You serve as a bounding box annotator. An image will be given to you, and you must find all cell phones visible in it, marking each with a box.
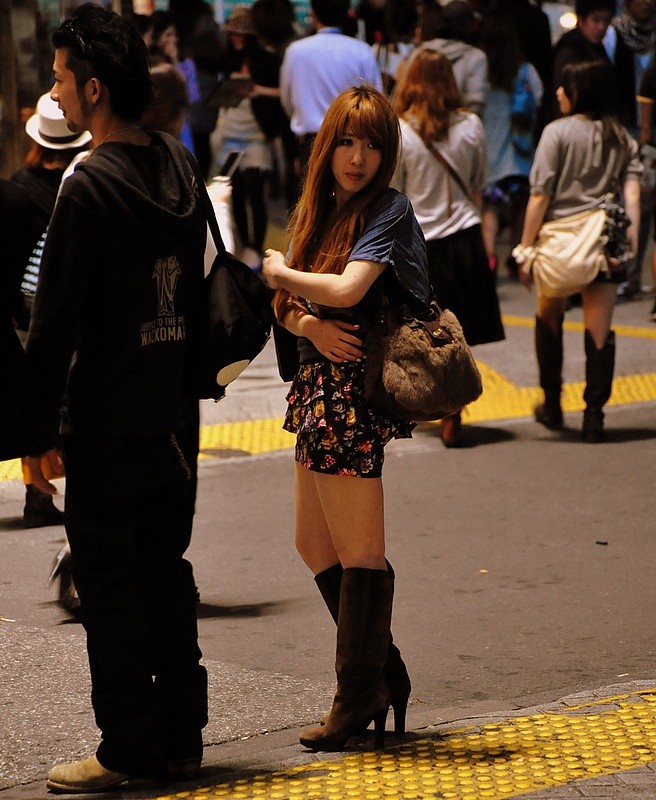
[212,150,244,182]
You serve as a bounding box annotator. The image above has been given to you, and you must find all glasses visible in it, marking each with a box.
[61,19,92,58]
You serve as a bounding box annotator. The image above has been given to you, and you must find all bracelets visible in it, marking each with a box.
[513,243,533,263]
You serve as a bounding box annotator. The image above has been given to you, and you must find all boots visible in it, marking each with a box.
[582,327,616,439]
[297,560,393,748]
[534,312,564,426]
[319,557,411,749]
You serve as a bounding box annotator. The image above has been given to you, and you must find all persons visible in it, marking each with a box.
[389,45,505,447]
[0,0,300,791]
[351,0,656,325]
[261,81,429,750]
[280,0,382,209]
[512,55,645,441]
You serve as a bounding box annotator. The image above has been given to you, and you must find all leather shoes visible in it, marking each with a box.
[47,751,198,792]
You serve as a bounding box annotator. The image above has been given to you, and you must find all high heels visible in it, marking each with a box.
[47,547,81,618]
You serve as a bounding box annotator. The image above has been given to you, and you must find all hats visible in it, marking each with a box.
[25,92,93,149]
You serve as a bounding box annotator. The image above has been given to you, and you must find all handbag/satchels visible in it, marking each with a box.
[181,146,273,401]
[532,211,609,298]
[511,63,540,157]
[365,192,483,421]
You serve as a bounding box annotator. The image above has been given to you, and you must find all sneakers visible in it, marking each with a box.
[441,420,461,445]
[22,497,67,528]
